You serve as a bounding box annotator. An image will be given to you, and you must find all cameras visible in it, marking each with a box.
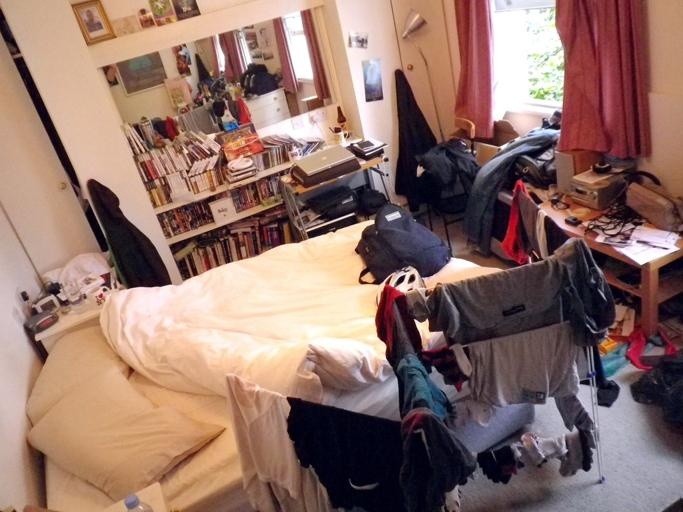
[565,215,582,226]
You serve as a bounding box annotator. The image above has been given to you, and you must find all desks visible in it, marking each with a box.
[536,193,683,343]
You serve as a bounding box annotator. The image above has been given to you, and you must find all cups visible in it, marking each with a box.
[85,285,112,309]
[333,128,349,144]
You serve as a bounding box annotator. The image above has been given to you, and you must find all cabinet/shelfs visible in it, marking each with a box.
[155,158,298,247]
[243,86,292,132]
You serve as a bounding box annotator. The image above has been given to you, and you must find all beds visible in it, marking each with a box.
[42,222,507,512]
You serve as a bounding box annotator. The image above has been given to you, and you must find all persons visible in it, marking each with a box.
[82,8,103,33]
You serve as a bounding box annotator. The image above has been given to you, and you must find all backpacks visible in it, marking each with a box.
[355,204,451,284]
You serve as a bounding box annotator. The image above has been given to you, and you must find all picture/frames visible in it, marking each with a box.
[110,51,170,98]
[72,1,119,46]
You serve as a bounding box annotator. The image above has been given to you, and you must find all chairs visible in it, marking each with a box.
[429,118,479,258]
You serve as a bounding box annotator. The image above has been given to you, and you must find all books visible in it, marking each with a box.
[122,115,327,206]
[158,203,291,281]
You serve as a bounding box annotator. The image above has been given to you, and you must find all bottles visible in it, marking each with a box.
[20,290,35,319]
[336,105,351,138]
[61,272,87,314]
[124,494,154,512]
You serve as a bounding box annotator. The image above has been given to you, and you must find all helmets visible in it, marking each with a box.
[376,266,425,307]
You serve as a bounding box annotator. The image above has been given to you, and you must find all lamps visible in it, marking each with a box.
[401,8,446,144]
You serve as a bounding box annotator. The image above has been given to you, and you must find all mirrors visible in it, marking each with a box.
[103,0,340,134]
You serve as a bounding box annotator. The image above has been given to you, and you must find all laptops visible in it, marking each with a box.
[294,144,356,176]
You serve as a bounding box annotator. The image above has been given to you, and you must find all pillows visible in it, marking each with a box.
[25,320,230,506]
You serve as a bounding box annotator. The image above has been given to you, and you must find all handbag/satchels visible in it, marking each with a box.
[358,188,388,216]
[515,154,549,190]
[625,180,683,231]
